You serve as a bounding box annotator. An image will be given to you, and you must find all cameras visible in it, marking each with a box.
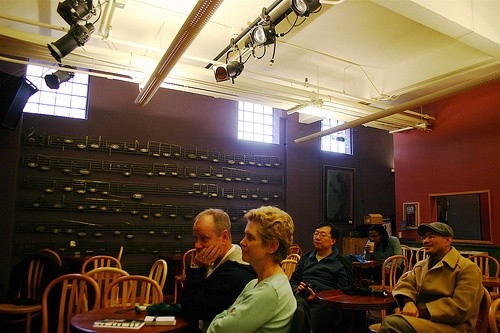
[298,282,317,299]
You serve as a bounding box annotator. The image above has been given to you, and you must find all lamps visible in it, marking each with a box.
[47,20,94,63]
[213,43,244,85]
[249,15,275,46]
[289,0,322,18]
[45,63,77,90]
[56,0,96,26]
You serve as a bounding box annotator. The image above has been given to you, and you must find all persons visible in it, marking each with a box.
[176,208,258,321]
[361,224,405,286]
[289,222,356,333]
[206,205,297,333]
[379,222,483,333]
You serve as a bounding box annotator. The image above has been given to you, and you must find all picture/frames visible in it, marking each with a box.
[428,189,494,245]
[323,165,354,224]
[402,202,420,229]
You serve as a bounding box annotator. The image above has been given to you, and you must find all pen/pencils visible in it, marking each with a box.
[153,315,157,322]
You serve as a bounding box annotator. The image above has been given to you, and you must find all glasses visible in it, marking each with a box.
[420,234,448,242]
[313,230,332,239]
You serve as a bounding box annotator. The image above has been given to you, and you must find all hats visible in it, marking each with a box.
[417,222,454,237]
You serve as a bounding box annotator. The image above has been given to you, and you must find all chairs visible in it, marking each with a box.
[0,245,500,333]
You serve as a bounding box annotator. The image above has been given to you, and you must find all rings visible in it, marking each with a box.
[202,255,206,259]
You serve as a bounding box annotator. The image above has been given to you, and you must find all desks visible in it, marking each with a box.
[351,260,378,288]
[314,288,398,333]
[70,306,186,333]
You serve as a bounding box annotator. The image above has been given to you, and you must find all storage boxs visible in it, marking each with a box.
[364,214,383,224]
